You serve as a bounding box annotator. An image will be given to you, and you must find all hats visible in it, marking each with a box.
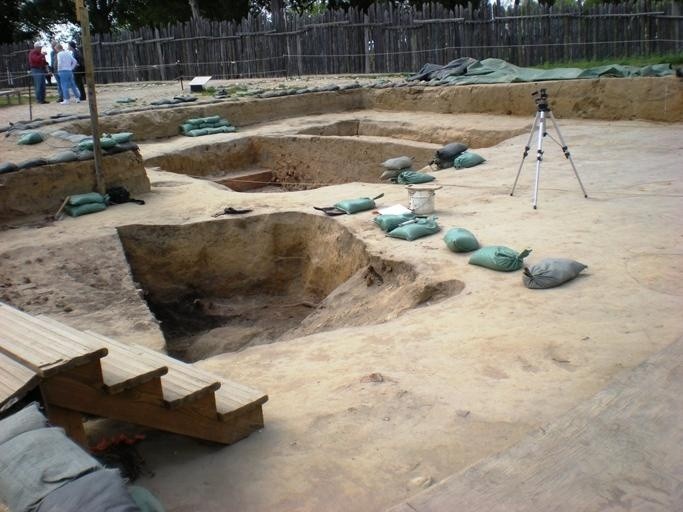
[33,42,44,48]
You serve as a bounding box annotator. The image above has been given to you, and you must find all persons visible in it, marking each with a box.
[27,41,51,105]
[54,43,81,106]
[48,41,71,103]
[66,41,86,101]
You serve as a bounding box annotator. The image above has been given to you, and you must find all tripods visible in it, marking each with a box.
[510,88,587,210]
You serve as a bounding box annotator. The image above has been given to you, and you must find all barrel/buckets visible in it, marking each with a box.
[408,188,436,215]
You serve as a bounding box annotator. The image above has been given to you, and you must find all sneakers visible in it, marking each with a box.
[36,97,86,106]
